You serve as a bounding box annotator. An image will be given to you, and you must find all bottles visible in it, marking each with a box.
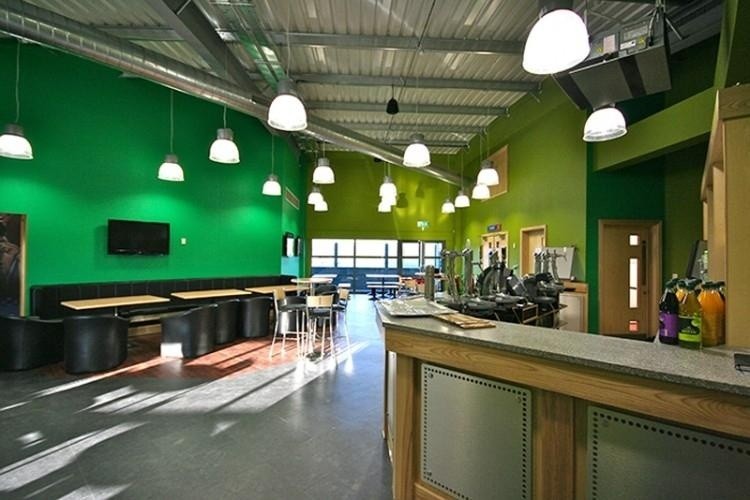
[698,284,706,305]
[710,281,724,345]
[699,286,718,347]
[424,265,435,301]
[719,280,725,342]
[658,281,677,345]
[675,280,688,301]
[677,283,703,352]
[673,277,677,292]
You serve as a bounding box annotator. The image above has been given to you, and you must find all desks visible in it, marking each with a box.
[364,273,442,299]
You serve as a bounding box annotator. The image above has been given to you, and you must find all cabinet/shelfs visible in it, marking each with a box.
[698,83,750,350]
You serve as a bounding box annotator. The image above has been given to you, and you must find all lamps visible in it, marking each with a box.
[521,0,591,75]
[582,103,627,142]
[0,37,33,160]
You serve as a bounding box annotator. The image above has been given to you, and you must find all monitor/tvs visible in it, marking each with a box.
[285,231,297,258]
[107,218,171,257]
[297,235,304,257]
